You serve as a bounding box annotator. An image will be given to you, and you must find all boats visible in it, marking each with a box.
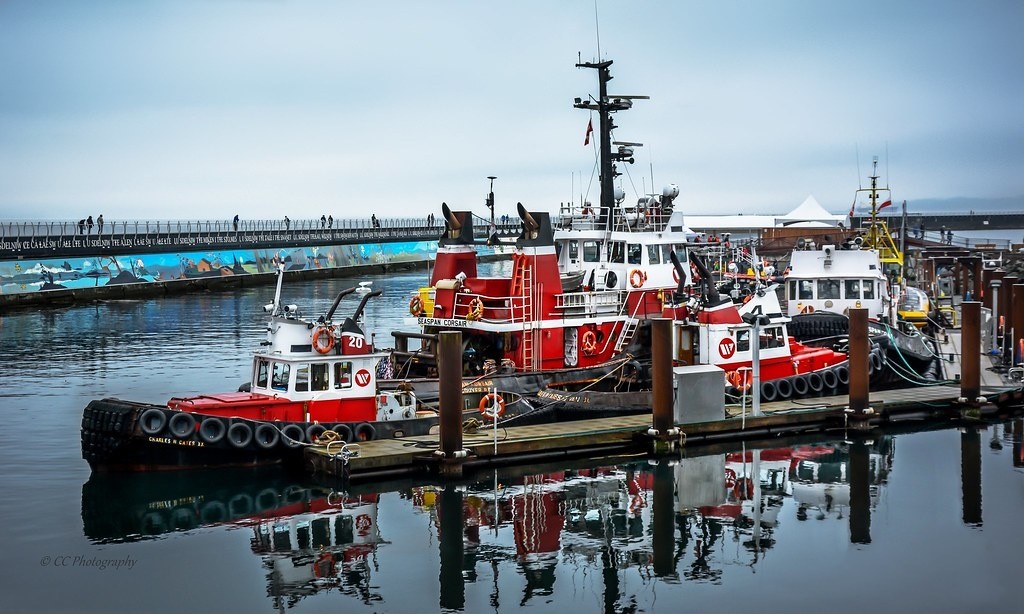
[82,44,931,471]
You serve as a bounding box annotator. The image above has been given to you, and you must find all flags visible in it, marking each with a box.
[585,117,594,146]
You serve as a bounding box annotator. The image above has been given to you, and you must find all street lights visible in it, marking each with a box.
[487,176,497,222]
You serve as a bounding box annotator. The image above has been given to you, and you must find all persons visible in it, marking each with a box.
[371,214,380,228]
[427,213,434,227]
[233,215,239,231]
[501,214,510,225]
[321,215,333,229]
[77,215,104,234]
[284,216,290,230]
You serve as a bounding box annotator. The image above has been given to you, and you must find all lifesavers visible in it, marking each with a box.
[639,208,650,223]
[479,393,506,422]
[409,295,426,317]
[137,407,377,450]
[139,482,336,536]
[312,327,336,355]
[734,476,755,500]
[468,299,485,322]
[582,331,596,355]
[760,341,887,401]
[313,552,338,581]
[629,270,645,289]
[733,366,753,391]
